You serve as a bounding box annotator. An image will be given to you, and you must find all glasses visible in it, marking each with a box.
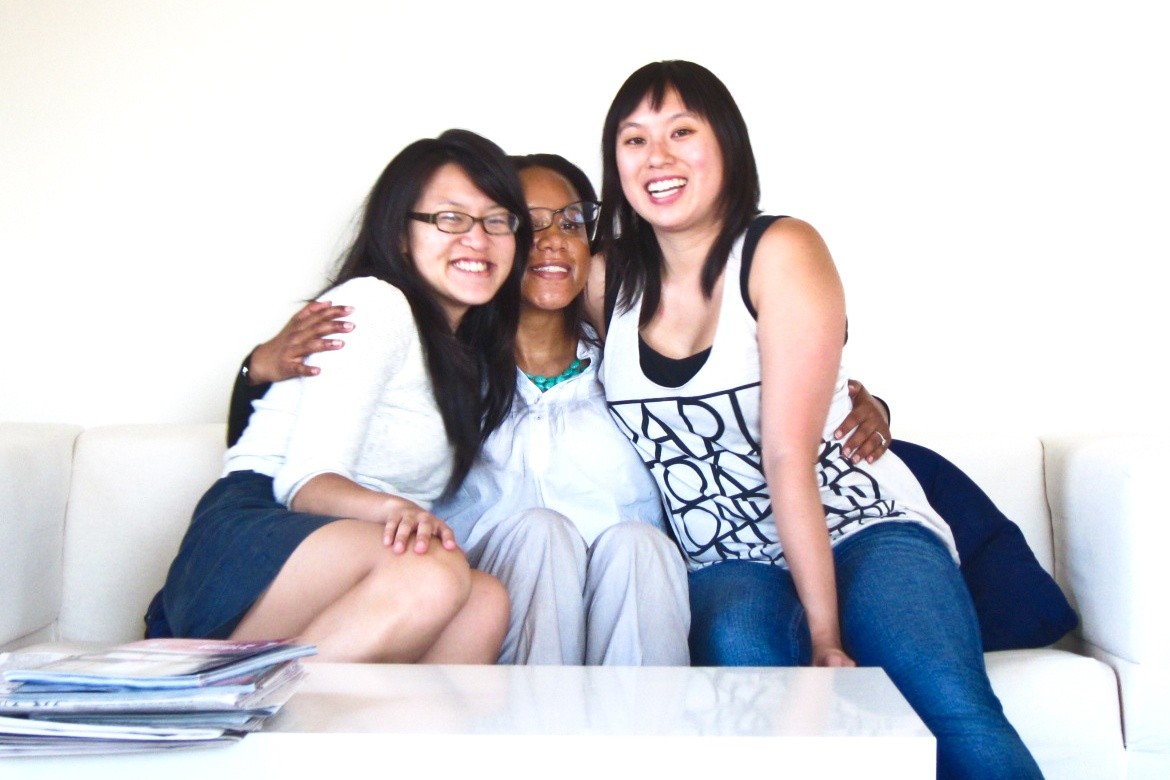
[529,199,604,233]
[409,211,520,233]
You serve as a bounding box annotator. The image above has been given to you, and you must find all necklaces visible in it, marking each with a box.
[523,359,588,392]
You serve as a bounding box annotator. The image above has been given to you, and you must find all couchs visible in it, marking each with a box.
[0,412,1170,780]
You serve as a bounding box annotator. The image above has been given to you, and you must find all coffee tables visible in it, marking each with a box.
[0,664,937,780]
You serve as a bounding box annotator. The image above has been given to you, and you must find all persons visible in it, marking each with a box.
[143,128,535,665]
[225,154,892,780]
[579,58,1046,780]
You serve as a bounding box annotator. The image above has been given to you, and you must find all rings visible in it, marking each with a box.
[877,430,890,456]
